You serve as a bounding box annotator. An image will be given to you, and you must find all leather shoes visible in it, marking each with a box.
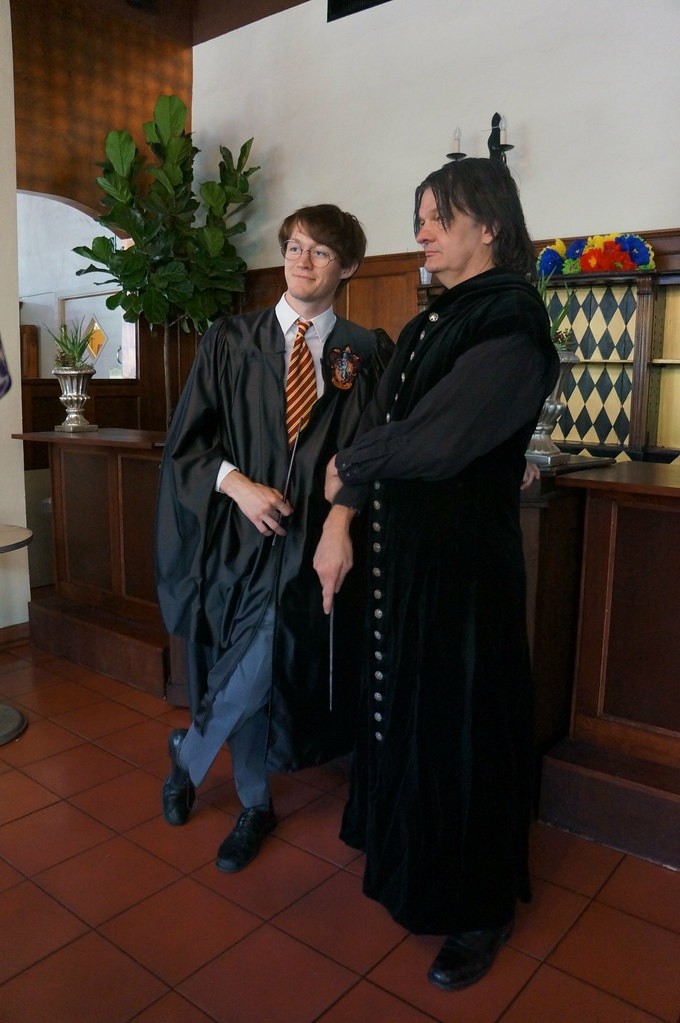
[426,914,514,992]
[215,798,278,873]
[162,729,196,825]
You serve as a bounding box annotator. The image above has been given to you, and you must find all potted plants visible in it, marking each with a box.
[43,315,100,433]
[523,264,582,467]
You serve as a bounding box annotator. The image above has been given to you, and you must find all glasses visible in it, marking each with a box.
[280,239,340,268]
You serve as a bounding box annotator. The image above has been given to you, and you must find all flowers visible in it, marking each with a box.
[535,232,657,276]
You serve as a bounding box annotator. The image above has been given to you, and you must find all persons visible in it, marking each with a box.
[158,206,543,873]
[277,157,561,994]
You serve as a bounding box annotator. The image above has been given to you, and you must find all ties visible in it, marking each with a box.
[285,319,319,451]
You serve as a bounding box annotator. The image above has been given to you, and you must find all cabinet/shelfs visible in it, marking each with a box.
[536,459,679,875]
[13,428,617,754]
